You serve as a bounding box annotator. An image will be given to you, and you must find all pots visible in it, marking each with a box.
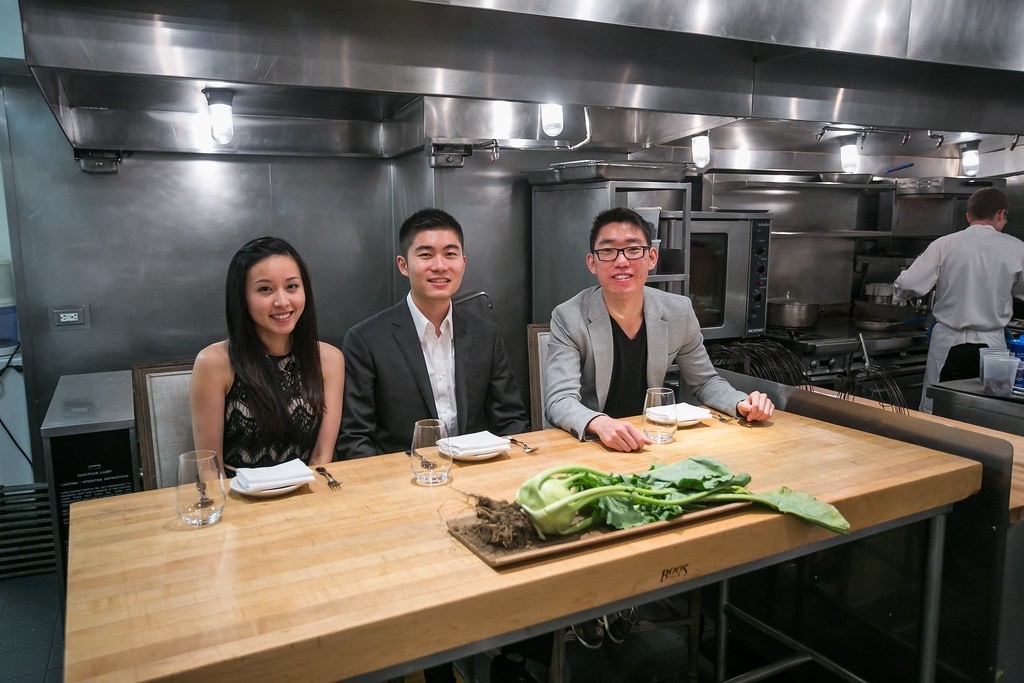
[853,318,927,331]
[768,290,824,329]
[851,301,927,323]
[819,163,914,184]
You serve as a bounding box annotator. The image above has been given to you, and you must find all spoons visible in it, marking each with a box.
[195,474,213,507]
[406,450,434,468]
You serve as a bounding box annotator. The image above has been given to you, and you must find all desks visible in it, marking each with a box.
[62,402,979,683]
[41,368,136,641]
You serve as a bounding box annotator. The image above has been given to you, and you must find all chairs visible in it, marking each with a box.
[527,323,706,682]
[132,359,202,491]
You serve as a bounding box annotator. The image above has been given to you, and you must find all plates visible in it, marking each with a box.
[439,446,503,461]
[230,476,305,497]
[677,420,701,427]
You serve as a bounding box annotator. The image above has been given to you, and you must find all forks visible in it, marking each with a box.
[710,412,732,422]
[315,467,342,487]
[507,437,540,453]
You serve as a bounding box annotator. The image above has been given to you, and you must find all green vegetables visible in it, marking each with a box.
[512,456,851,541]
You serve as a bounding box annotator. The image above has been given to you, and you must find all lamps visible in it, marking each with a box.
[956,139,982,179]
[839,135,860,174]
[541,101,562,138]
[692,130,712,168]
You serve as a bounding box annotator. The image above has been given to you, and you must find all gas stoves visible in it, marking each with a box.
[765,313,932,365]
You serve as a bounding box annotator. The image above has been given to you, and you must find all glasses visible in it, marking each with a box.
[591,245,652,261]
[997,210,1010,224]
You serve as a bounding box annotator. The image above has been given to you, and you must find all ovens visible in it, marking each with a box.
[661,210,773,342]
[810,366,925,410]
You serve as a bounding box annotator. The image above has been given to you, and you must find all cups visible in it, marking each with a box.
[980,348,1009,384]
[411,419,453,486]
[983,356,1020,396]
[632,207,661,240]
[177,450,226,527]
[642,388,678,444]
[649,240,661,274]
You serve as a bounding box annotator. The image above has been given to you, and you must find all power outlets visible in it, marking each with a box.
[48,304,93,331]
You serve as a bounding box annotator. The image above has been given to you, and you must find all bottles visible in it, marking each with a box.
[1010,340,1024,389]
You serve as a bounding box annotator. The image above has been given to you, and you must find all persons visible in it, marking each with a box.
[335,208,531,465]
[543,207,775,648]
[189,237,345,482]
[892,188,1024,415]
